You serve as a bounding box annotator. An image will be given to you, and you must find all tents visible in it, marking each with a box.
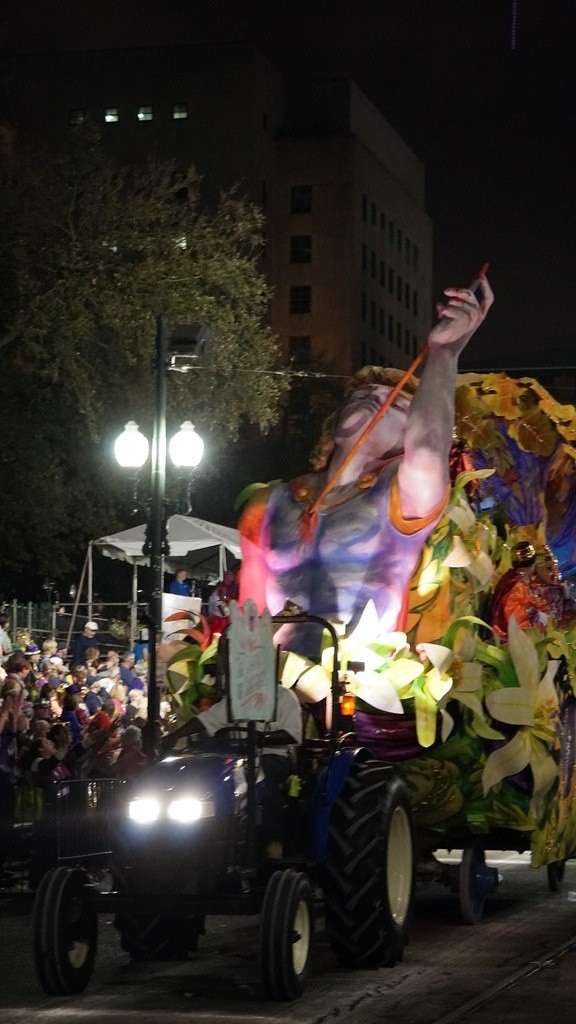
[89,514,243,621]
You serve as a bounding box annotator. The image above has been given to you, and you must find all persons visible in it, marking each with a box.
[0,569,240,893]
[488,540,576,646]
[450,433,494,514]
[240,276,494,718]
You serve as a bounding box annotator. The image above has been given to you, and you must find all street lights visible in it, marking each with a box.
[106,312,211,763]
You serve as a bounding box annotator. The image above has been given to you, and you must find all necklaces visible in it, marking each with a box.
[295,463,382,507]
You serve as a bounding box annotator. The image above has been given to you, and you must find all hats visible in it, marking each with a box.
[22,646,42,656]
[84,621,100,630]
[65,683,84,696]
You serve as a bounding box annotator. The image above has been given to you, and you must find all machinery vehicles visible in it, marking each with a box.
[31,596,416,998]
